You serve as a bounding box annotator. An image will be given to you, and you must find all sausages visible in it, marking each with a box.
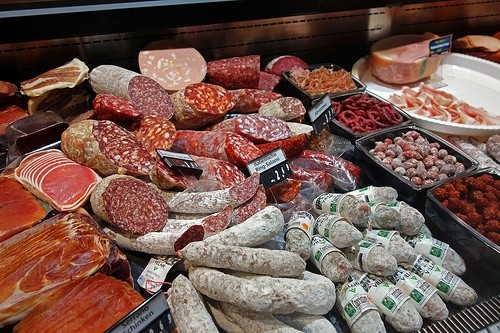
[14,55,478,333]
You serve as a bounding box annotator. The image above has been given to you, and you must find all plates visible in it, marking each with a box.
[351,52,500,137]
